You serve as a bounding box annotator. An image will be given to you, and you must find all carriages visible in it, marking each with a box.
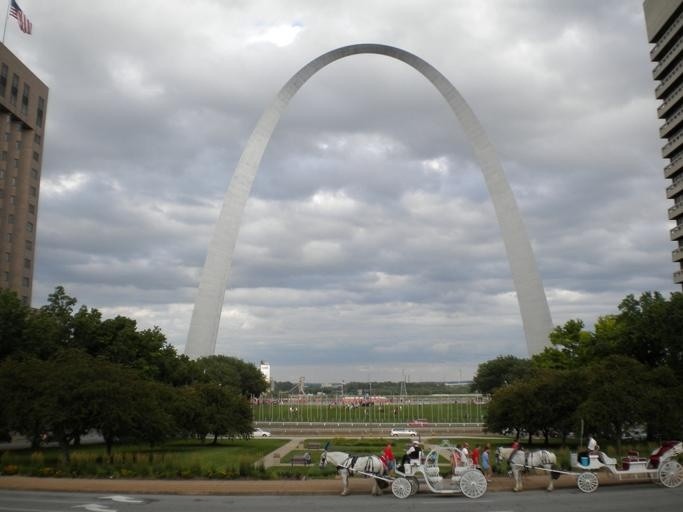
[318,447,488,499]
[494,441,683,492]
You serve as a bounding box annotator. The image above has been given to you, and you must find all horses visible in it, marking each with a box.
[318,450,389,496]
[495,446,557,491]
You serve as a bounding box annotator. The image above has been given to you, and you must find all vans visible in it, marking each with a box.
[391,428,417,437]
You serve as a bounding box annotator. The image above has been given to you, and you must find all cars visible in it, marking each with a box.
[407,418,428,428]
[252,428,271,437]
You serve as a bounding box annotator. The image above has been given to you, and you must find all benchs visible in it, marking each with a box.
[649,441,673,465]
[598,451,617,469]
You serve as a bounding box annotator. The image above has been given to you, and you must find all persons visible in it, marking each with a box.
[588,445,603,464]
[383,442,395,468]
[396,440,421,468]
[428,439,520,481]
[586,433,598,461]
